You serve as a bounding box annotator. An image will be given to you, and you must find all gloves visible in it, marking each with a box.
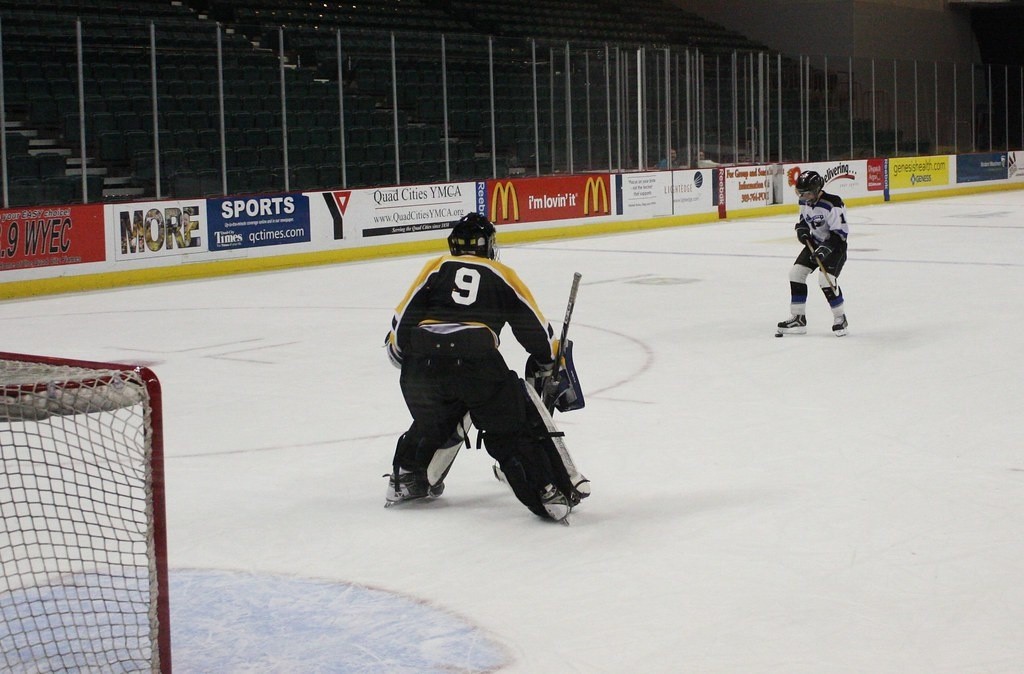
[797,232,811,245]
[543,374,562,397]
[801,251,820,268]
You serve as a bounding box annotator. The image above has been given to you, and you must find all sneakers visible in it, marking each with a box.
[385,467,445,509]
[541,485,584,525]
[777,314,807,333]
[832,313,848,337]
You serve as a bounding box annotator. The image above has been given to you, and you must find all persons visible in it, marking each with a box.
[656,147,679,169]
[382,212,590,526]
[777,171,849,337]
[691,149,705,168]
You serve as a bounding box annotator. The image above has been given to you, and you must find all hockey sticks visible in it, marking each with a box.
[806,239,839,297]
[491,271,583,483]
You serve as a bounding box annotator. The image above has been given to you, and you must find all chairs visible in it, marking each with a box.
[0,0,929,208]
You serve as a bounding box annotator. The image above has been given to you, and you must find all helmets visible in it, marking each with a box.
[448,213,495,259]
[795,170,824,197]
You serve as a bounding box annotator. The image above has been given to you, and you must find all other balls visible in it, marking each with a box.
[775,334,783,337]
